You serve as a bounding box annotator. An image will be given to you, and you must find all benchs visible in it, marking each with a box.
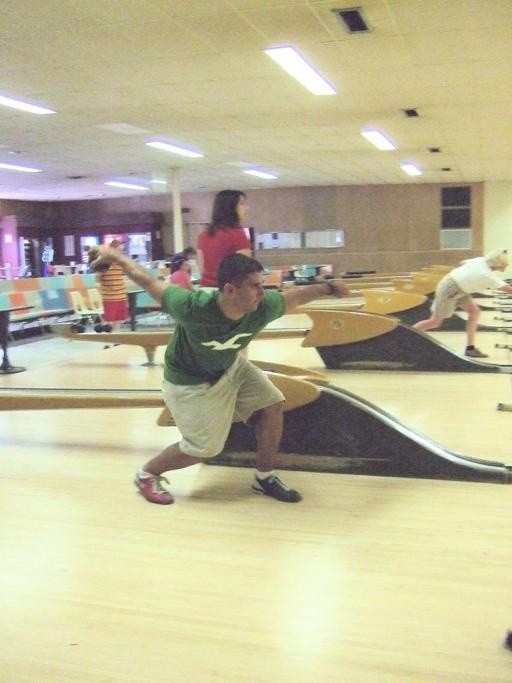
[8,308,75,342]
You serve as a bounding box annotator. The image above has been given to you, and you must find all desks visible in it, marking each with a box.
[0,306,34,374]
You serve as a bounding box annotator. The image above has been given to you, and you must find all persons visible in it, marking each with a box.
[171,259,196,292]
[86,240,353,506]
[410,249,512,357]
[194,187,253,287]
[171,245,198,279]
[93,239,129,349]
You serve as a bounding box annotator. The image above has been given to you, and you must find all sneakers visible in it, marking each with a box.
[252,474,302,502]
[136,469,172,504]
[465,346,488,357]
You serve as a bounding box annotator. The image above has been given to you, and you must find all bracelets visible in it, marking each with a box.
[326,281,336,294]
